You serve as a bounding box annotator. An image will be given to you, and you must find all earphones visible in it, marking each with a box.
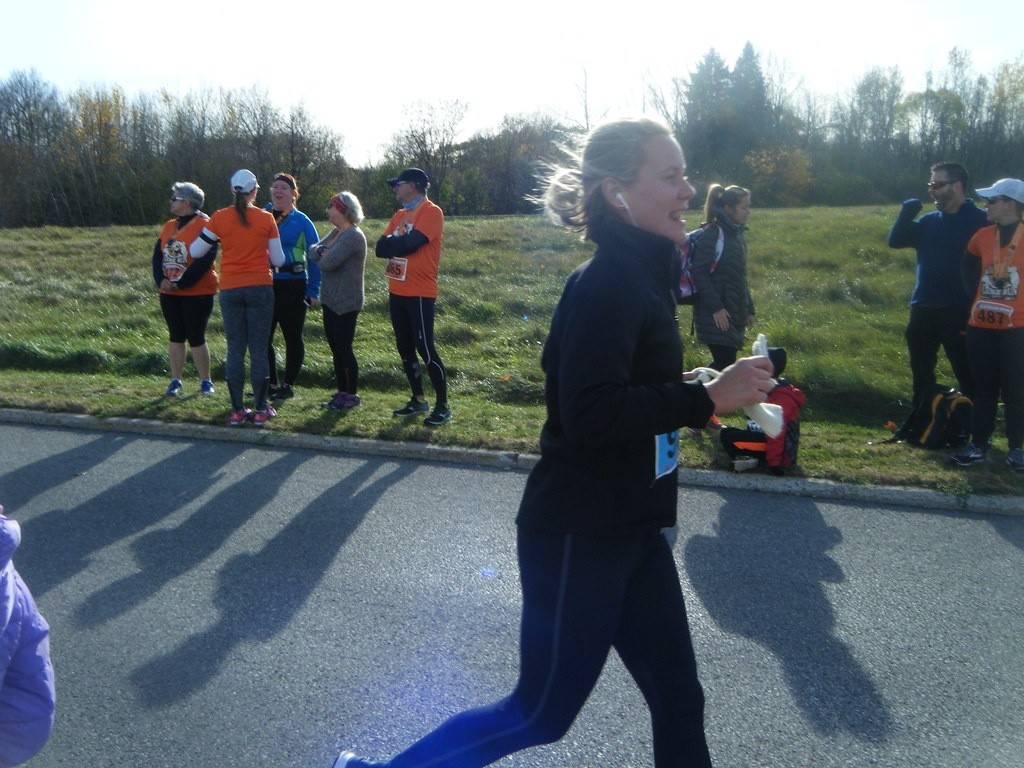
[615,192,630,211]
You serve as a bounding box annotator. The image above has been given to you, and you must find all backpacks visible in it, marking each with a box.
[893,382,973,449]
[671,221,725,305]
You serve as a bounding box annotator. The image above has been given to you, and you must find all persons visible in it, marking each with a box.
[889,162,995,409]
[0,507,54,767]
[687,183,755,435]
[331,119,773,768]
[248,173,321,399]
[190,169,285,426]
[308,191,367,410]
[717,346,806,474]
[152,181,218,399]
[375,168,453,426]
[949,177,1024,472]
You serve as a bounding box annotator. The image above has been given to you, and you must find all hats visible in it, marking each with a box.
[231,169,260,193]
[386,167,429,187]
[974,178,1024,205]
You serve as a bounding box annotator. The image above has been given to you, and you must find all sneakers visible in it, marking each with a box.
[1006,447,1024,471]
[230,407,252,425]
[950,448,985,466]
[279,384,294,400]
[320,393,360,409]
[423,403,453,424]
[392,396,429,418]
[255,405,277,425]
[201,380,215,393]
[166,379,182,397]
[247,384,280,399]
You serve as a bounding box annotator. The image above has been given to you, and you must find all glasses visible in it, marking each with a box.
[927,177,963,190]
[327,203,337,209]
[170,195,185,202]
[987,196,1011,205]
[396,181,420,186]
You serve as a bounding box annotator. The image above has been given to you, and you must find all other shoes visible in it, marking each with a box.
[979,435,994,453]
[707,415,727,433]
[730,455,760,472]
[332,750,376,768]
